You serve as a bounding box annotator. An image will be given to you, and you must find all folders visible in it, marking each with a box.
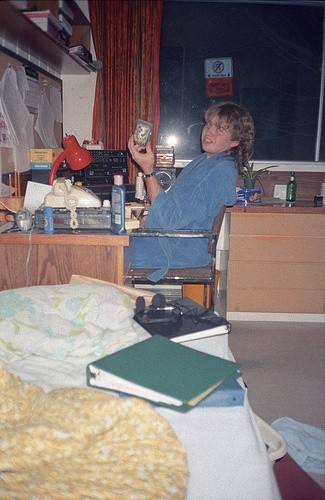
[84,334,245,414]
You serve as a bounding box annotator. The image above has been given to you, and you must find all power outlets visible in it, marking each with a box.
[274,184,287,199]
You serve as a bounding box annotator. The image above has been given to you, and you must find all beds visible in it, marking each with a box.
[0,283,281,500]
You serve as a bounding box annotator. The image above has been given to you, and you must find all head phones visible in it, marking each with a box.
[135,293,180,324]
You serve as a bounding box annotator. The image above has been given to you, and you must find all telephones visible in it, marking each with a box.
[42,178,100,208]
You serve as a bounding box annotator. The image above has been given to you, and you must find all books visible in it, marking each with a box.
[69,45,93,62]
[133,297,232,343]
[86,334,241,414]
[119,379,245,407]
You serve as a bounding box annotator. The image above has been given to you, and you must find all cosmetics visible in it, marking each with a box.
[44,207,54,233]
[111,175,127,235]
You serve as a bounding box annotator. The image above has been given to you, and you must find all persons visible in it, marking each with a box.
[124,102,256,283]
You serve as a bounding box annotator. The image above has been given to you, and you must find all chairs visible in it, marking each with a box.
[123,206,226,312]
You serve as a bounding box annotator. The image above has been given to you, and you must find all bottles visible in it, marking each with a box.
[135,172,146,199]
[286,177,297,202]
[111,175,127,234]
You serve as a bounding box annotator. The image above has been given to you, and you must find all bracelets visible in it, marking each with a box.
[145,171,155,177]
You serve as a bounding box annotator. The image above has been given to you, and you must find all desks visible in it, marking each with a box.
[225,199,325,323]
[0,229,129,291]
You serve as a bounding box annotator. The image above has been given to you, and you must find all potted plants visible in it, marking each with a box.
[244,161,279,196]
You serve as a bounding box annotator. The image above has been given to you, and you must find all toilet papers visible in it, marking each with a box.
[135,177,146,200]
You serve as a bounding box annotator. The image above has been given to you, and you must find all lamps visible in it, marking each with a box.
[48,132,93,186]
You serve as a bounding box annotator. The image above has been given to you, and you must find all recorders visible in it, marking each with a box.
[73,150,129,186]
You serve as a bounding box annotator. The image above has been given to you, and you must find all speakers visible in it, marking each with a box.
[154,167,176,192]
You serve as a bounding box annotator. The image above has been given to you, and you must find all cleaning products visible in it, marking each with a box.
[109,175,127,234]
